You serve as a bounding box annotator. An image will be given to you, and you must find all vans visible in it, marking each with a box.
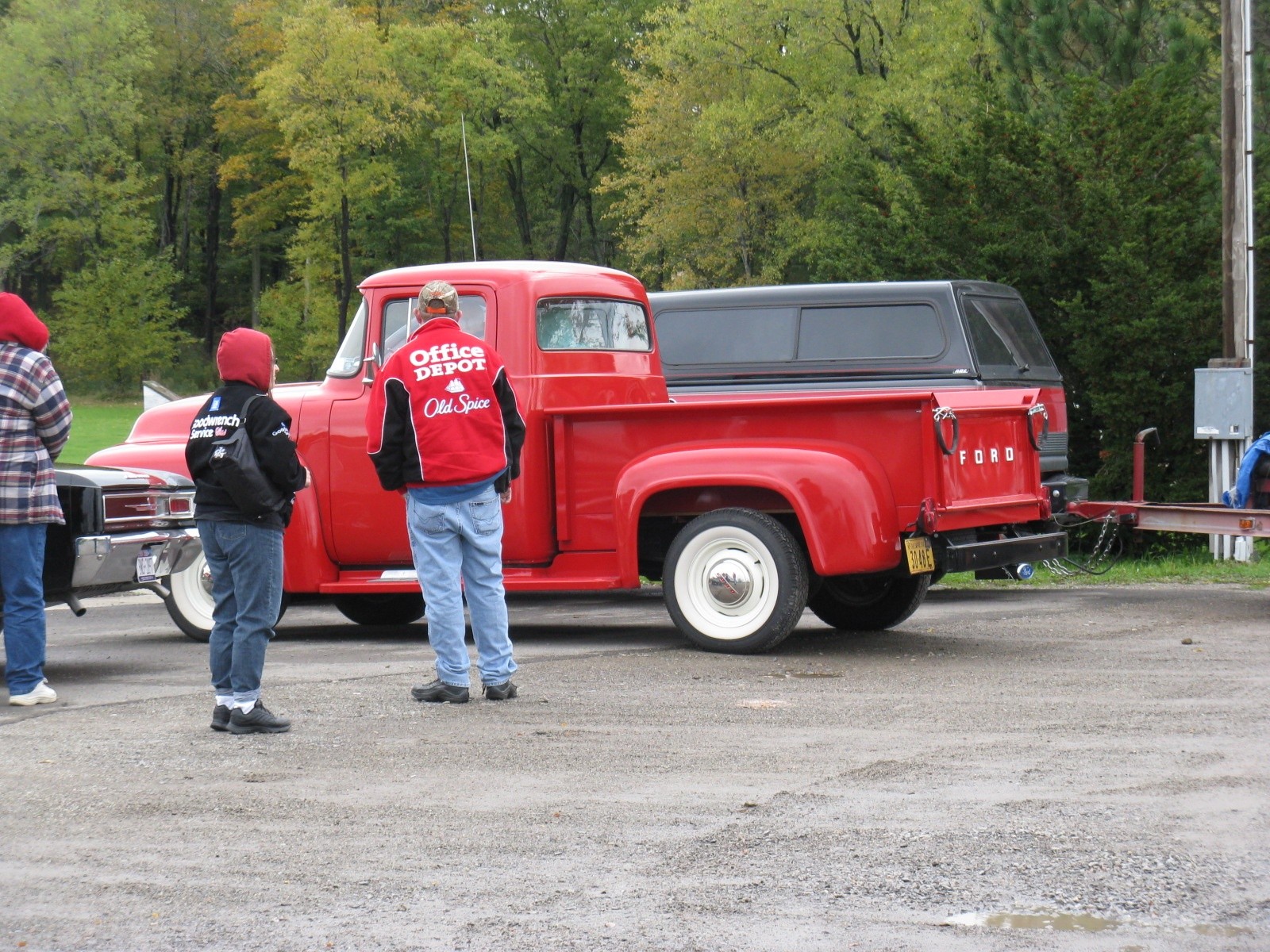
[379,279,1092,585]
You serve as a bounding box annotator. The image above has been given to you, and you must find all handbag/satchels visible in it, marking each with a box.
[209,394,297,522]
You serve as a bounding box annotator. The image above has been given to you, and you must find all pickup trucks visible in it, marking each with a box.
[83,260,1069,660]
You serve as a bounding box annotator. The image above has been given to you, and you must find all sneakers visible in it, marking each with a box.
[412,678,469,704]
[227,699,291,734]
[211,703,232,732]
[486,680,518,700]
[9,678,58,706]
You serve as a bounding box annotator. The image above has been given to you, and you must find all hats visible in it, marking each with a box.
[418,281,459,315]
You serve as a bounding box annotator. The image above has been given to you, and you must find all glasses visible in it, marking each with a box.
[271,357,278,364]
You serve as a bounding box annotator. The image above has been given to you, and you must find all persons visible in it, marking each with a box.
[366,281,526,702]
[185,327,311,735]
[0,291,73,707]
